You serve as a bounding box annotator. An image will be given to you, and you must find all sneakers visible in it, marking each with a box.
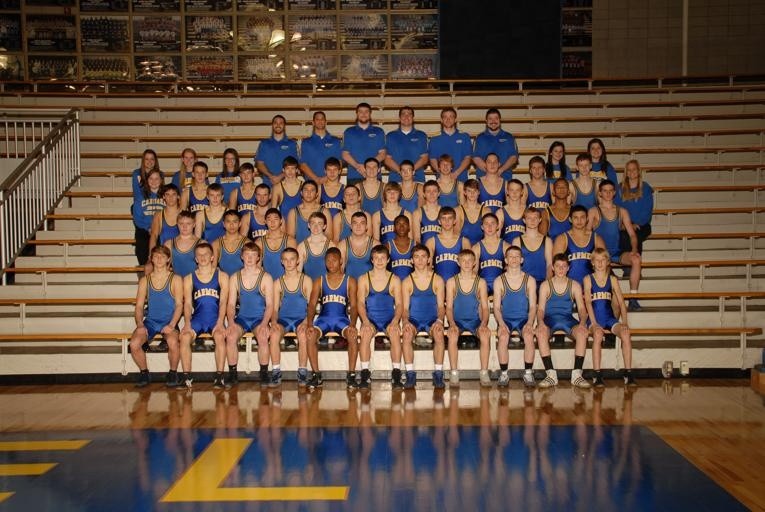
[522,370,536,388]
[628,300,642,311]
[432,371,446,389]
[176,373,194,390]
[297,369,309,387]
[404,371,417,389]
[571,369,591,388]
[307,371,324,389]
[345,372,359,390]
[592,372,606,388]
[166,371,177,387]
[391,369,404,390]
[449,370,460,389]
[225,372,239,390]
[538,369,559,387]
[496,370,510,388]
[359,369,372,390]
[268,369,283,389]
[259,370,270,388]
[213,372,225,389]
[134,372,152,388]
[479,370,492,388]
[623,372,637,387]
[622,269,631,278]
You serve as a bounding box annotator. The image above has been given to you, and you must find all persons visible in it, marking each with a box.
[404,247,447,391]
[395,159,423,212]
[131,169,167,263]
[424,206,472,282]
[583,246,638,388]
[383,215,420,281]
[587,138,618,185]
[286,179,333,243]
[510,206,553,282]
[272,156,305,220]
[307,246,359,389]
[148,183,183,259]
[229,162,260,214]
[493,245,538,388]
[132,148,164,203]
[428,107,473,182]
[341,102,386,186]
[385,106,428,185]
[453,179,491,244]
[586,178,644,312]
[212,210,252,275]
[300,111,343,183]
[551,205,606,286]
[472,108,519,182]
[254,115,297,185]
[333,186,372,245]
[316,156,346,215]
[353,157,386,215]
[470,213,512,300]
[172,147,209,191]
[478,153,508,214]
[567,153,601,210]
[370,181,414,241]
[357,245,404,389]
[253,207,297,279]
[544,141,573,183]
[523,155,554,211]
[194,183,229,244]
[496,179,530,243]
[446,248,493,389]
[295,212,338,281]
[240,183,273,241]
[215,148,245,203]
[337,211,381,280]
[225,242,272,388]
[178,242,228,389]
[130,245,182,388]
[145,210,209,277]
[434,154,465,208]
[613,159,652,277]
[412,179,443,245]
[536,252,592,389]
[268,247,308,388]
[177,161,212,215]
